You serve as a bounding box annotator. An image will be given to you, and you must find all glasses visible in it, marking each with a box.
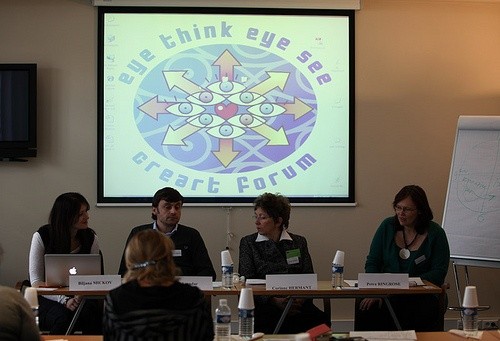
[252,216,269,221]
[395,206,417,212]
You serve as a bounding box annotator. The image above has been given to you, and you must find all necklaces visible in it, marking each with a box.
[399,226,421,259]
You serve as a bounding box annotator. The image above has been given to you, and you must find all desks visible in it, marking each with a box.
[36,281,443,336]
[31,330,500,341]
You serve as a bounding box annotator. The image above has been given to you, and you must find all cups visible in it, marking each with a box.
[237,288,255,309]
[461,286,479,308]
[220,250,234,267]
[332,250,345,266]
[24,287,41,307]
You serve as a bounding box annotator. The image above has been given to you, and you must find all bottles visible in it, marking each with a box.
[237,309,255,341]
[331,264,344,289]
[216,299,232,341]
[222,266,234,289]
[463,309,479,338]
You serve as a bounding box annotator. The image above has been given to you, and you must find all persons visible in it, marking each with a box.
[358,185,450,332]
[101,230,215,341]
[119,187,217,281]
[238,194,331,335]
[29,192,100,335]
[0,285,41,341]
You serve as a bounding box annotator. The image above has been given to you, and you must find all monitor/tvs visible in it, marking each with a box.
[0,63,37,157]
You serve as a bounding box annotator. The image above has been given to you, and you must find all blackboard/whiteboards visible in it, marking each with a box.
[441,115,500,269]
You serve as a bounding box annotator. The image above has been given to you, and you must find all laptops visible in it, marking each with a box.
[45,254,101,288]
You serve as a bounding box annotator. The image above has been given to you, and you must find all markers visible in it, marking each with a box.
[252,333,264,340]
